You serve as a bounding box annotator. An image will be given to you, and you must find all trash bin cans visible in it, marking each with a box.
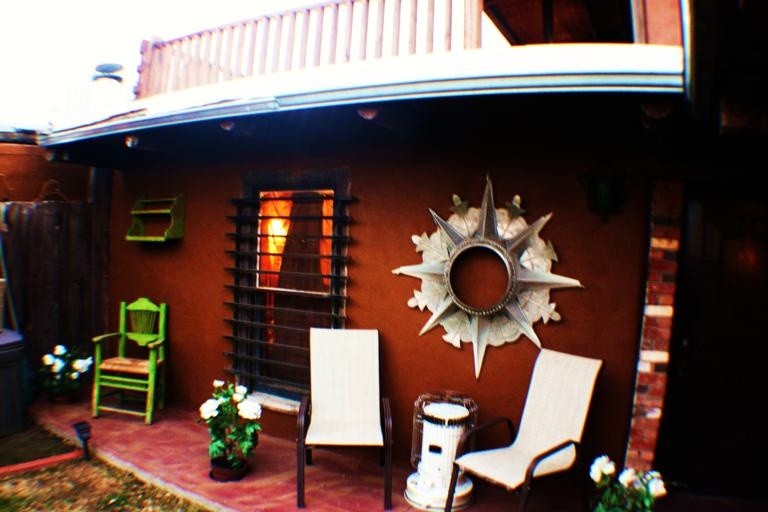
[0,329,26,439]
[403,391,479,512]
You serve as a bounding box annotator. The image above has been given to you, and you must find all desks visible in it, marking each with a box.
[402,388,482,510]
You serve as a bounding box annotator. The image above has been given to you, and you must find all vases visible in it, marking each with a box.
[596,500,625,511]
[207,456,246,483]
[51,378,86,401]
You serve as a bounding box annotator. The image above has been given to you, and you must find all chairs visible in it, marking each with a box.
[90,298,174,426]
[296,324,395,512]
[441,346,603,512]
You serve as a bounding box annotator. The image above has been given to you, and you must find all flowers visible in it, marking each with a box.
[41,344,93,383]
[194,376,263,469]
[589,452,666,512]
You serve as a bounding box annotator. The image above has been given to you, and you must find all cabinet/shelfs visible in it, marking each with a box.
[126,195,185,243]
